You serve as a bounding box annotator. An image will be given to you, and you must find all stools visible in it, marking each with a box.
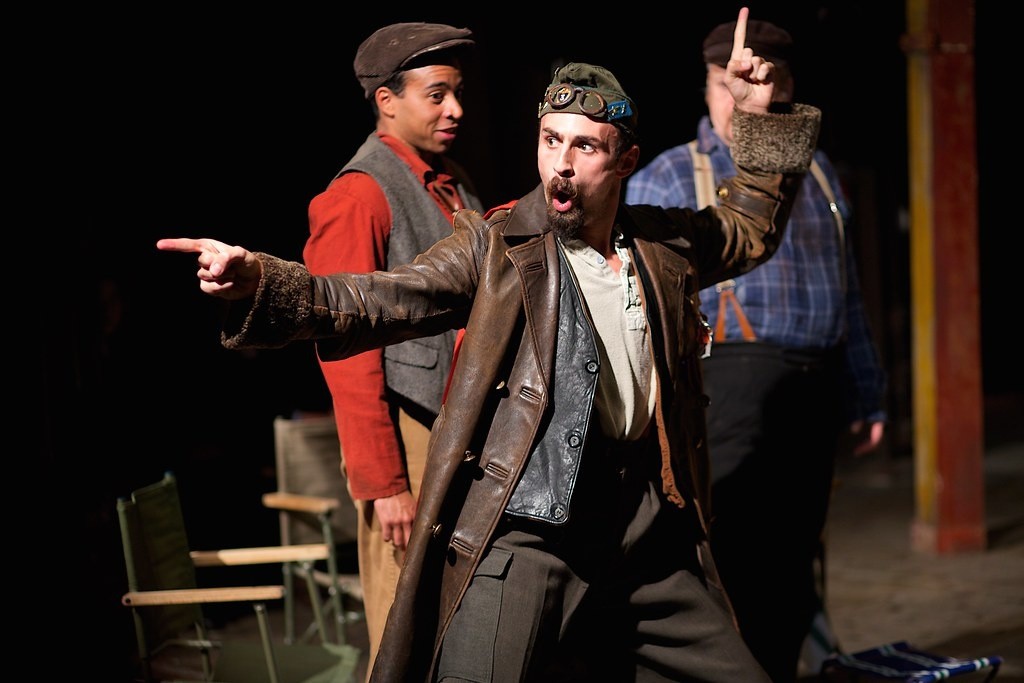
[819,640,1004,683]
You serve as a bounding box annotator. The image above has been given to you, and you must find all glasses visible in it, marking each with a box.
[542,84,633,133]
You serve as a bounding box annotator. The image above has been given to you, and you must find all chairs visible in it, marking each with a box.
[117,416,365,683]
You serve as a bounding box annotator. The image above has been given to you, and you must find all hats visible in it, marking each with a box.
[354,22,475,98]
[535,58,638,134]
[705,20,795,69]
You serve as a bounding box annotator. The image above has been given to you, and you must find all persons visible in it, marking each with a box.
[627,22,891,683]
[303,23,486,683]
[158,6,819,683]
